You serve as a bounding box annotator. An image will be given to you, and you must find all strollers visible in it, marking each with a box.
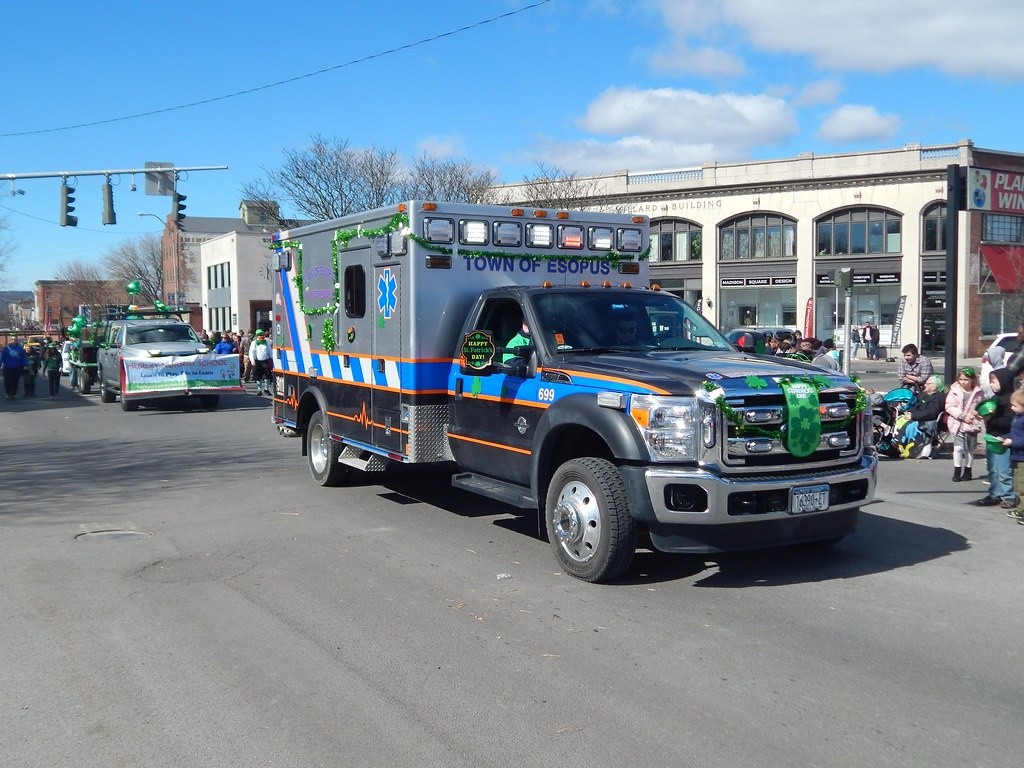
[872,384,919,453]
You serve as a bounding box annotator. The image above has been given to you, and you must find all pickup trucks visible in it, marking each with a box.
[96,319,220,411]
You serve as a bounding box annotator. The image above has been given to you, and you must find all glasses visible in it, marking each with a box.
[925,383,932,385]
[800,344,811,348]
[221,337,227,339]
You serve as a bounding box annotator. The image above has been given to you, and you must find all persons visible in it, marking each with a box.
[862,321,879,360]
[850,325,862,360]
[974,321,1024,524]
[42,343,63,400]
[945,366,987,482]
[501,316,558,368]
[865,388,891,436]
[0,335,30,401]
[22,345,42,398]
[876,373,948,459]
[896,343,933,395]
[763,330,842,371]
[195,327,273,396]
[605,311,641,346]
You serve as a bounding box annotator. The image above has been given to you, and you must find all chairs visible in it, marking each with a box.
[501,309,554,357]
[603,311,644,345]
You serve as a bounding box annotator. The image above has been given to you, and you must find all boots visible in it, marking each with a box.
[960,466,971,480]
[952,467,962,482]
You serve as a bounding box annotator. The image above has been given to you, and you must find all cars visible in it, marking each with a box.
[61,341,72,375]
[24,335,57,356]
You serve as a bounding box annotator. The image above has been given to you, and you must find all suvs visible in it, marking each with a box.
[979,333,1019,392]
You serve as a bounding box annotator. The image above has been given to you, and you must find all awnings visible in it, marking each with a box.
[979,245,1024,294]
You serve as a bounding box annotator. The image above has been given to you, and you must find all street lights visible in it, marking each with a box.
[137,213,177,310]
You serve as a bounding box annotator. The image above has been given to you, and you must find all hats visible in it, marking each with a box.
[866,320,870,323]
[48,343,57,349]
[255,329,265,337]
[975,398,1002,417]
[983,434,1009,455]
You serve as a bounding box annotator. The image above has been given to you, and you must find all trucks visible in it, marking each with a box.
[272,200,879,584]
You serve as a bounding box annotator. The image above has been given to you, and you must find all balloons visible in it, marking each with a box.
[67,315,87,335]
[125,280,141,295]
[154,300,172,312]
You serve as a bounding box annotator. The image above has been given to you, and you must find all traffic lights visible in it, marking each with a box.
[172,193,187,222]
[60,186,78,227]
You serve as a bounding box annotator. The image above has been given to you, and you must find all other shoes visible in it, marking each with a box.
[1000,494,1020,509]
[978,496,1001,505]
[264,389,272,396]
[1017,518,1024,525]
[257,391,262,396]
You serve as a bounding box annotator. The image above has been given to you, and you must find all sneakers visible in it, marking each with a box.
[1006,510,1023,519]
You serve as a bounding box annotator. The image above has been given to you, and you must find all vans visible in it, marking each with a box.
[724,328,794,350]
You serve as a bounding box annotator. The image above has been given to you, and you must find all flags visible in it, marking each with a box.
[690,298,702,341]
[804,298,813,339]
[891,296,906,345]
